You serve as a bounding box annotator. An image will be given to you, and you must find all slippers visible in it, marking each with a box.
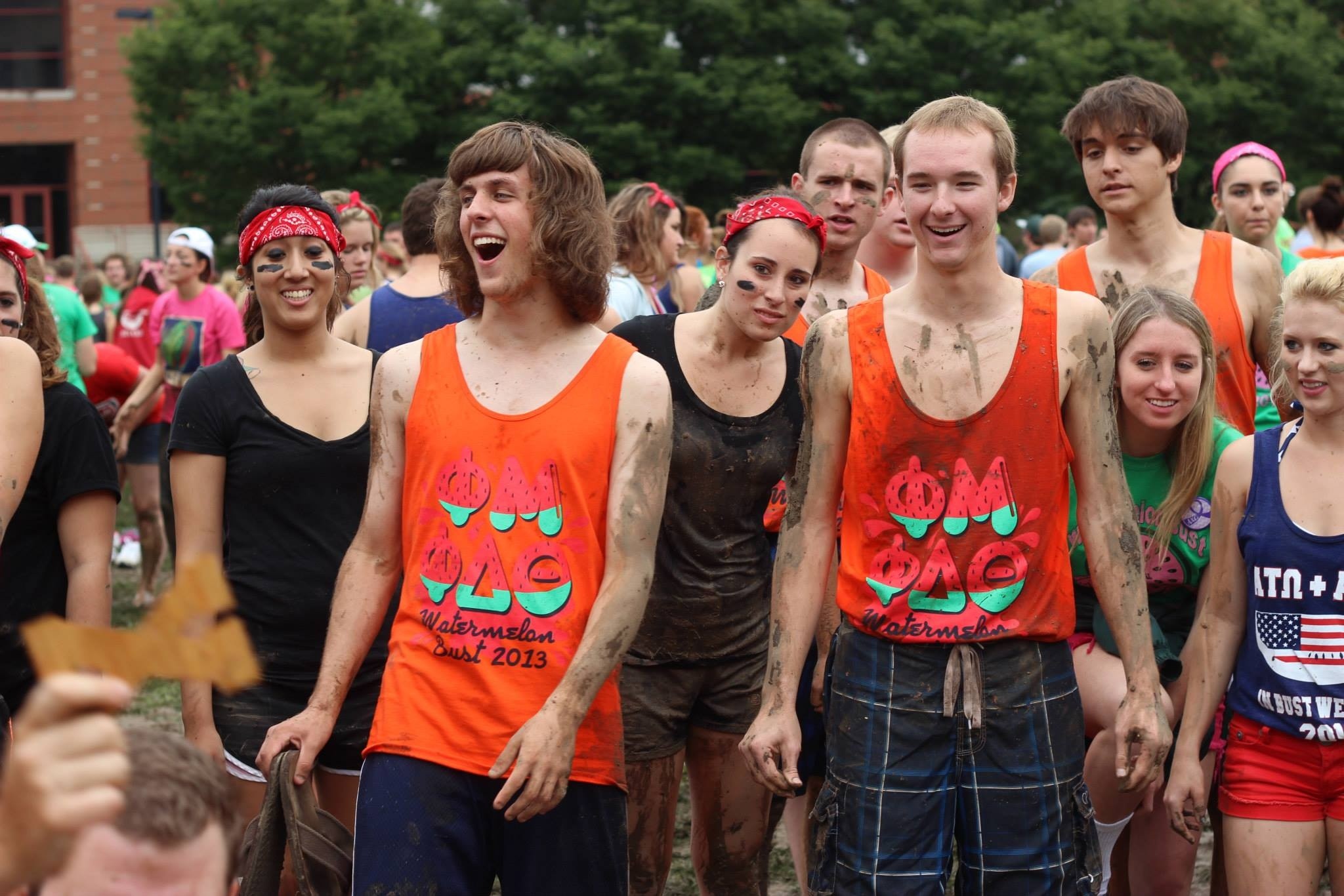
[235,752,287,896]
[279,749,354,896]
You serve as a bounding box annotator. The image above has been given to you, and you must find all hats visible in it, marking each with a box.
[167,227,215,283]
[1025,214,1042,239]
[1,223,49,253]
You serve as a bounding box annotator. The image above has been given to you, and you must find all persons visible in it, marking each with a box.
[598,74,1344,896]
[1,120,674,896]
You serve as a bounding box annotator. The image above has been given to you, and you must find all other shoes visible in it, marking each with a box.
[114,537,141,568]
